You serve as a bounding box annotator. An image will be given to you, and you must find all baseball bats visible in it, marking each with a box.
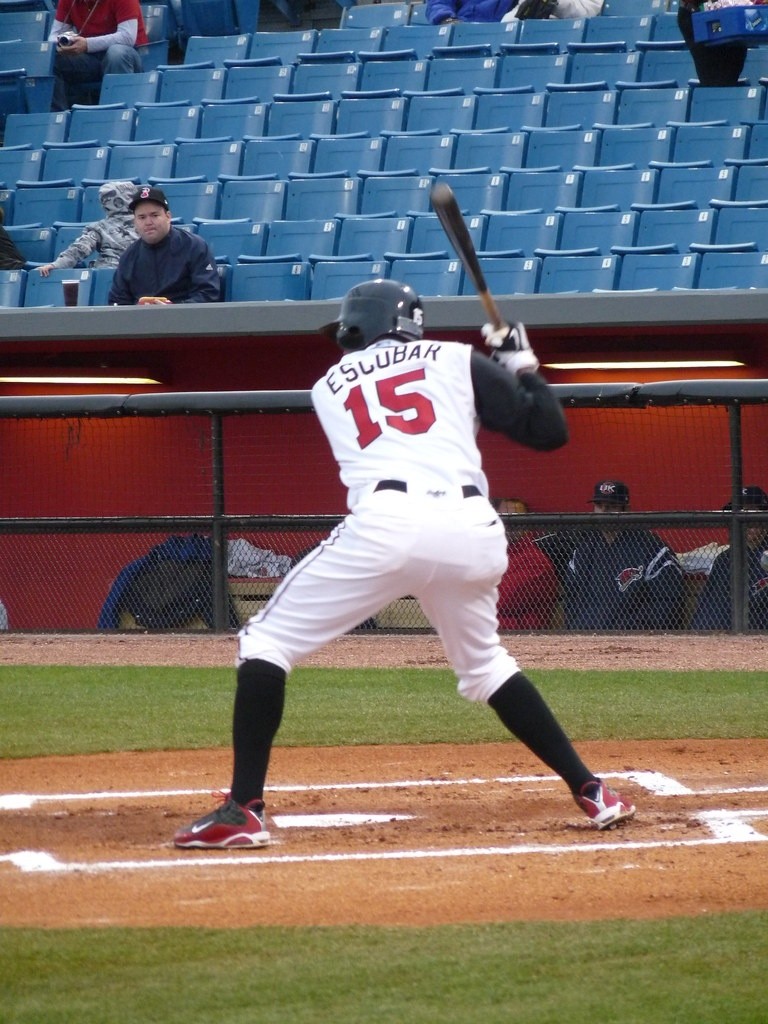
[431,180,504,330]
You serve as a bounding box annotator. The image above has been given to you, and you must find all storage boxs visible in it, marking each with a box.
[691,4,768,48]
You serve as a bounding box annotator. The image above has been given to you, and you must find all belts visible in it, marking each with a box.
[375,479,483,499]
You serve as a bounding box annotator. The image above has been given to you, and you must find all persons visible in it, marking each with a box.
[0,208,25,270]
[537,481,684,629]
[495,498,557,630]
[677,0,768,86]
[109,186,223,305]
[426,0,519,25]
[49,0,149,112]
[690,486,768,629]
[34,181,140,278]
[173,279,637,847]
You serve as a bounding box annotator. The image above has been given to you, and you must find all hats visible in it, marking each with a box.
[723,485,767,509]
[587,479,630,506]
[129,187,169,210]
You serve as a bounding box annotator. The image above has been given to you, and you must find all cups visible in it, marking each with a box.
[62,279,80,306]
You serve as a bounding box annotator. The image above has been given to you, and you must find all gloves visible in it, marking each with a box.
[481,320,539,375]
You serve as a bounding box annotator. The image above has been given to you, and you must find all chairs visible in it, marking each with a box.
[0,0,768,308]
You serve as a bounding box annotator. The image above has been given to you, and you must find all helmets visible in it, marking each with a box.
[318,278,425,350]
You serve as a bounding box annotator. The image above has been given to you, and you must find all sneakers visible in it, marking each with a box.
[571,776,636,829]
[172,788,270,850]
